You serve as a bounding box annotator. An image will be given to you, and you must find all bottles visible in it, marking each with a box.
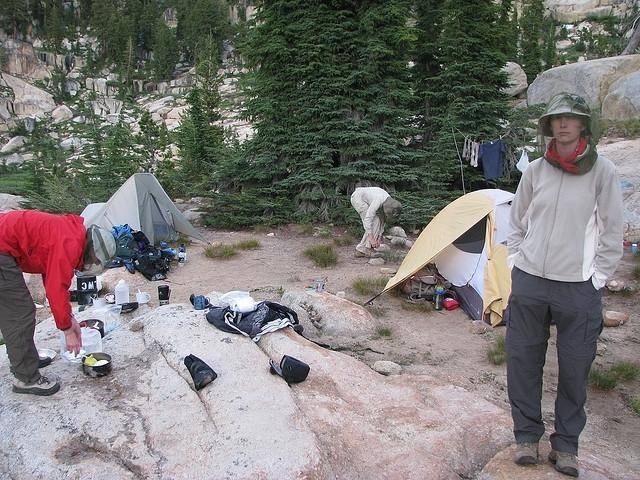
[433,284,445,311]
[168,231,180,247]
[176,243,186,267]
[631,243,638,257]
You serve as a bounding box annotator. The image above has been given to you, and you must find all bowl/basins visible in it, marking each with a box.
[37,348,57,361]
[72,319,112,378]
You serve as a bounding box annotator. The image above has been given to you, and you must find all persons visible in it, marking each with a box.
[350,186,402,257]
[504,93,624,478]
[0,209,117,396]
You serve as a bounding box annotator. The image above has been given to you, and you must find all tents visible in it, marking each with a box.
[364,188,516,327]
[80,173,210,247]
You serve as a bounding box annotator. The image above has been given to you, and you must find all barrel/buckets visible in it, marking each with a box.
[177,244,187,267]
[60,327,103,362]
[79,319,104,338]
[158,285,169,300]
[77,275,97,305]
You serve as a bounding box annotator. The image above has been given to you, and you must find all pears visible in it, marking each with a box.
[85,357,97,365]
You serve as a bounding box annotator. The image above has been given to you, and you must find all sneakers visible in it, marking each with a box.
[11,376,61,397]
[354,249,374,257]
[513,441,540,467]
[37,355,51,367]
[546,447,580,479]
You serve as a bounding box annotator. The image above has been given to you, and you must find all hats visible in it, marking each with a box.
[382,196,404,227]
[91,223,117,269]
[538,91,593,137]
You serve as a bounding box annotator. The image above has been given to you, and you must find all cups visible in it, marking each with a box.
[159,240,167,249]
[194,295,211,310]
[315,278,326,294]
[158,285,170,306]
[92,292,151,313]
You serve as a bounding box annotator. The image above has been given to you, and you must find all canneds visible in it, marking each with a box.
[433,294,443,310]
